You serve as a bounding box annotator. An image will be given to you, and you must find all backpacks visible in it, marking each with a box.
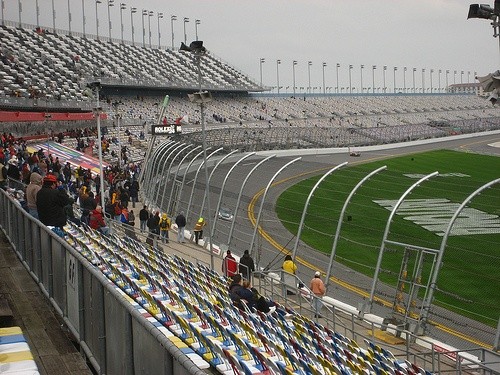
[160,218,169,229]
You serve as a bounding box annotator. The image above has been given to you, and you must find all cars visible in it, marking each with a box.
[216,207,234,222]
[350,151,360,157]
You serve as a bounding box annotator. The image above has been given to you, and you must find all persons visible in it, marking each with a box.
[35,175,69,226]
[0,121,149,205]
[228,273,276,316]
[193,217,208,244]
[65,194,188,253]
[221,250,237,280]
[99,86,500,146]
[238,249,256,285]
[0,24,107,103]
[309,270,326,318]
[283,255,297,296]
[24,172,44,221]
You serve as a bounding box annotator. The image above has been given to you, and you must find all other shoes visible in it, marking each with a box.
[287,292,295,295]
[315,315,322,318]
[266,305,276,316]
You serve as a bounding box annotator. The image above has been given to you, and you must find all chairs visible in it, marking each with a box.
[47,204,432,375]
[0,327,40,375]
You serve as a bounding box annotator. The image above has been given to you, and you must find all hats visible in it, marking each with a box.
[43,174,58,182]
[315,271,321,276]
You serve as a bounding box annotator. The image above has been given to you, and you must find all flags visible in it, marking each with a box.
[96,0,201,25]
[259,58,479,76]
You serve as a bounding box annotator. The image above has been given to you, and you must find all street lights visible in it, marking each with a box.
[85,82,108,216]
[112,100,123,166]
[180,40,214,270]
